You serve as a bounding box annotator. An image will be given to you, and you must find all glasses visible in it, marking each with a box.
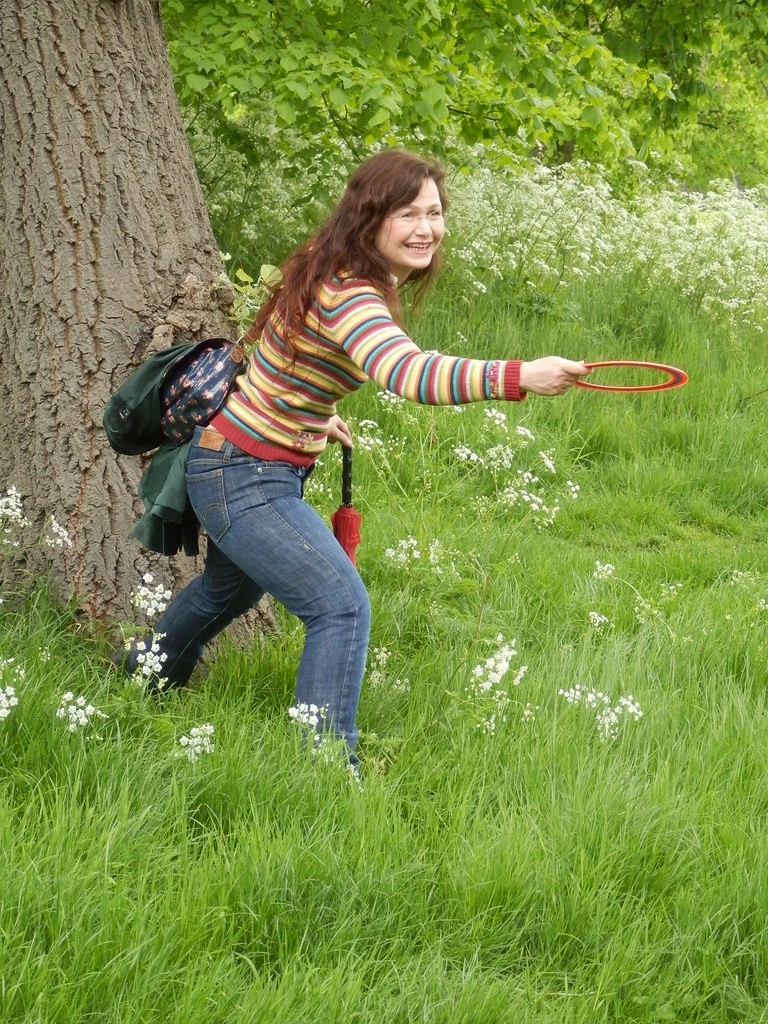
[389,209,443,227]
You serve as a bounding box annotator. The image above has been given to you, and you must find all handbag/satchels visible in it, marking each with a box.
[160,344,243,441]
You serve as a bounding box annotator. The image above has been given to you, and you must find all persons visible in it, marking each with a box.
[127,150,590,764]
